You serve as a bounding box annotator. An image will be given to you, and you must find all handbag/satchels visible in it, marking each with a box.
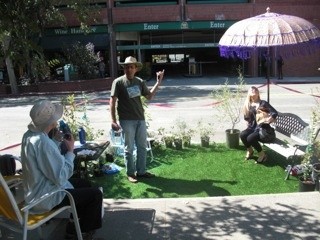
[258,127,275,143]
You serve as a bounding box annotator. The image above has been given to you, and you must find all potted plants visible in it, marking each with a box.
[173,132,182,149]
[180,119,196,146]
[197,119,215,146]
[299,142,320,191]
[161,136,173,148]
[212,65,249,148]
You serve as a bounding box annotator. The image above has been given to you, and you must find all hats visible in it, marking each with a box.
[120,56,143,72]
[28,100,63,133]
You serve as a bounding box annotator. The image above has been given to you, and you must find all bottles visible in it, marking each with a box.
[78,128,85,144]
[59,120,70,139]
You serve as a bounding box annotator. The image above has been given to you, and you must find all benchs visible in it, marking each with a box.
[259,110,311,181]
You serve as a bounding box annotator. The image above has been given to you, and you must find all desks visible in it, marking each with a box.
[71,141,110,179]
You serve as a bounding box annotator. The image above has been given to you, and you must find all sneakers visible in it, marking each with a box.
[66,222,94,240]
[255,155,266,163]
[245,149,254,161]
[136,172,155,178]
[128,175,138,183]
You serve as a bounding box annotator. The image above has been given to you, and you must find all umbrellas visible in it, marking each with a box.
[219,7,320,103]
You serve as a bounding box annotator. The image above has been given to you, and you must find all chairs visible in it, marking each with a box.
[0,154,84,240]
[110,126,155,162]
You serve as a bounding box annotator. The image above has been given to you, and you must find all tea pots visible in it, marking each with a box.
[1,155,21,177]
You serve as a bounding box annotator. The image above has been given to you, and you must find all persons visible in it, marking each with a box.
[21,101,103,240]
[276,56,284,79]
[240,86,278,163]
[110,56,165,183]
